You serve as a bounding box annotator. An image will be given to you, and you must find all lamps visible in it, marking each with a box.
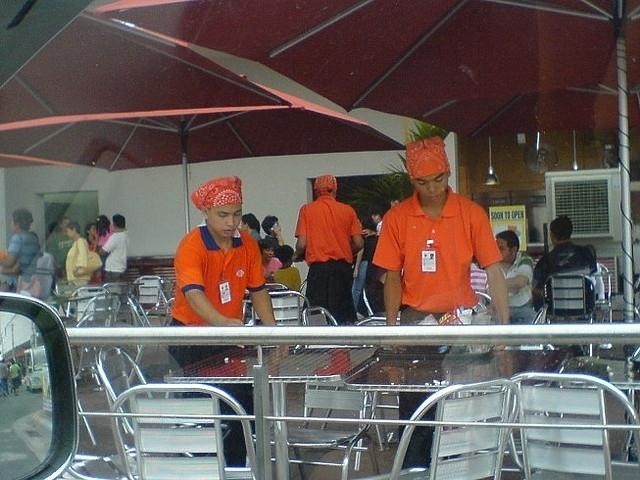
[484,136,499,185]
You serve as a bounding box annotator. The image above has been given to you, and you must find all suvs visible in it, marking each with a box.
[22,346,47,391]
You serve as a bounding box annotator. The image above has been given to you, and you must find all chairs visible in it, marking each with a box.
[67,263,640,480]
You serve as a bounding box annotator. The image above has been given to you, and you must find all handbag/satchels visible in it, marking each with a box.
[73,251,103,278]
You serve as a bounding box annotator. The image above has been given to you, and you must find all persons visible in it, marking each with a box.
[1,206,127,302]
[292,175,363,324]
[533,215,597,318]
[469,256,489,306]
[353,199,403,317]
[240,213,301,295]
[490,229,534,326]
[372,137,513,470]
[166,175,289,467]
[0,357,26,401]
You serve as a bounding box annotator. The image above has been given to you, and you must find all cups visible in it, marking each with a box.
[455,310,472,325]
